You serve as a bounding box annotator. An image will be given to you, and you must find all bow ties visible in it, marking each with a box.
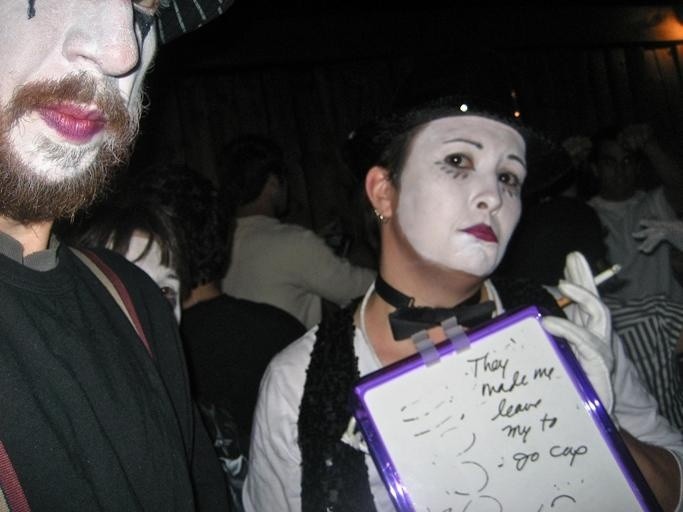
[389,301,497,342]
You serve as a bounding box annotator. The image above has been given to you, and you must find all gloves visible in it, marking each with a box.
[633,219,680,252]
[542,251,616,416]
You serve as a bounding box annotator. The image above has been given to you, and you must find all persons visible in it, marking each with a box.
[0,1,682,511]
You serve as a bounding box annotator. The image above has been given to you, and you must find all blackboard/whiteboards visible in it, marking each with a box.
[348,303,663,512]
